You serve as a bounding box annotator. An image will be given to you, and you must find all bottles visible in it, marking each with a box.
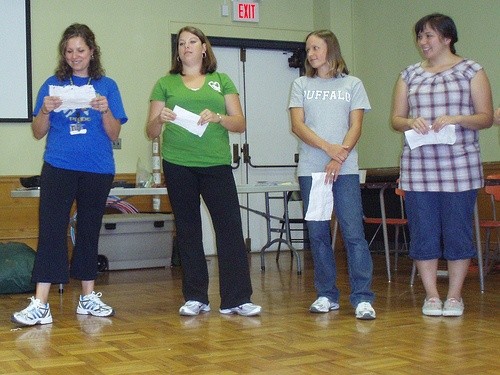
[152,136,162,187]
[152,194,162,213]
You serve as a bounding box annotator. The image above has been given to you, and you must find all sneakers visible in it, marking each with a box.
[178,300,211,316]
[354,301,376,319]
[443,298,464,316]
[421,297,443,316]
[76,312,111,333]
[309,296,340,312]
[76,291,115,316]
[14,324,51,350]
[12,296,53,325]
[219,302,262,316]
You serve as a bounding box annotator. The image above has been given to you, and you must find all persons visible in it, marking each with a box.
[390,13,495,318]
[10,23,126,325]
[146,25,264,316]
[289,29,378,319]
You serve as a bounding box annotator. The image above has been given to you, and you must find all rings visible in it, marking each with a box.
[442,121,445,123]
[331,172,335,175]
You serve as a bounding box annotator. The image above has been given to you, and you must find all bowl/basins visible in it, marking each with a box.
[21,175,43,188]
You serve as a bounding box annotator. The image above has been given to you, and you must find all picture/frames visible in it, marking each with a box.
[0,0,33,123]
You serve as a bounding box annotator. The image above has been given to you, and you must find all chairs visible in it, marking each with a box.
[364,179,408,272]
[479,173,500,277]
[275,192,310,263]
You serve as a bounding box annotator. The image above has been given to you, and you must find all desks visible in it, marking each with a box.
[11,182,301,295]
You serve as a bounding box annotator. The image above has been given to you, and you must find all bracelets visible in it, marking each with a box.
[101,107,110,114]
[40,108,49,114]
[217,111,223,122]
